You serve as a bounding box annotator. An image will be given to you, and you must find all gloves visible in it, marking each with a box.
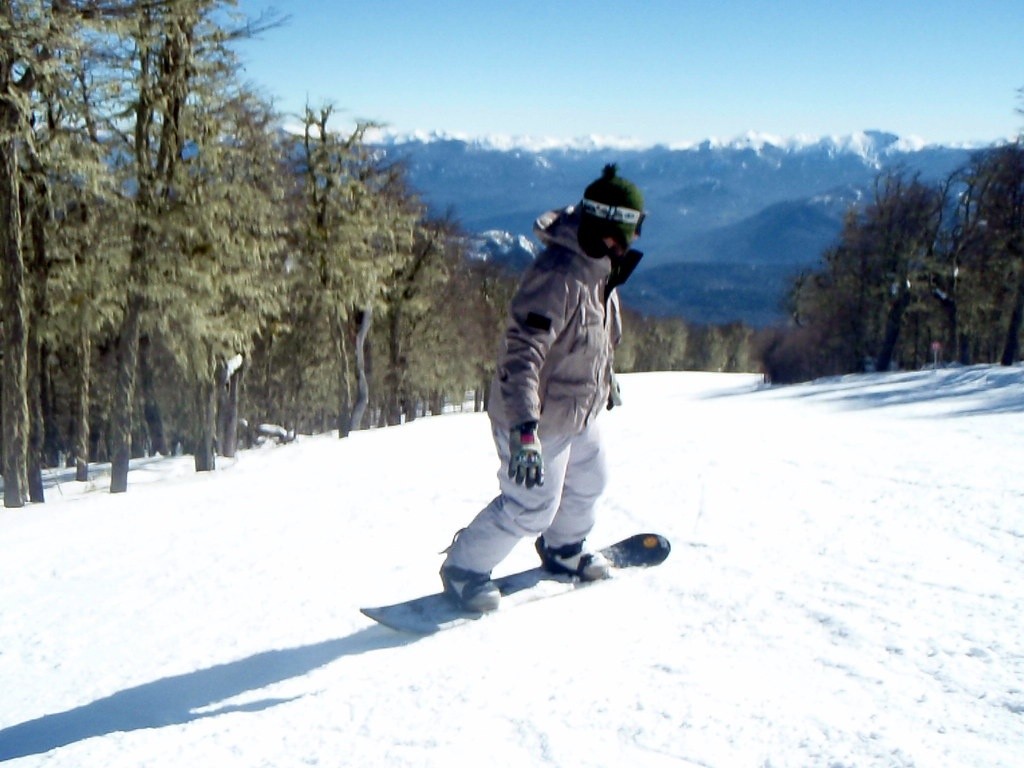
[508,424,544,489]
[606,379,622,410]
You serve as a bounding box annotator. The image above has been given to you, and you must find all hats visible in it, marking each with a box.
[582,162,642,246]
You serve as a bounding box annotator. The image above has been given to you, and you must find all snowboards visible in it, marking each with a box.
[358,532,672,634]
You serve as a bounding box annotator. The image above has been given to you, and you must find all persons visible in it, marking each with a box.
[440,164,646,610]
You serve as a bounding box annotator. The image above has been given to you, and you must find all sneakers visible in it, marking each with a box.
[542,542,608,578]
[439,559,500,610]
